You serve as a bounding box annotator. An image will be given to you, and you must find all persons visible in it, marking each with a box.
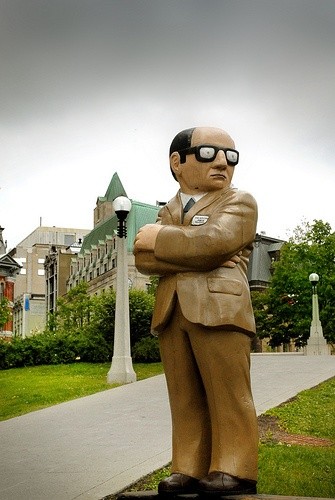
[132,127,260,496]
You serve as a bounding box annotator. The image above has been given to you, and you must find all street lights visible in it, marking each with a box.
[304,272,330,356]
[106,196,137,382]
